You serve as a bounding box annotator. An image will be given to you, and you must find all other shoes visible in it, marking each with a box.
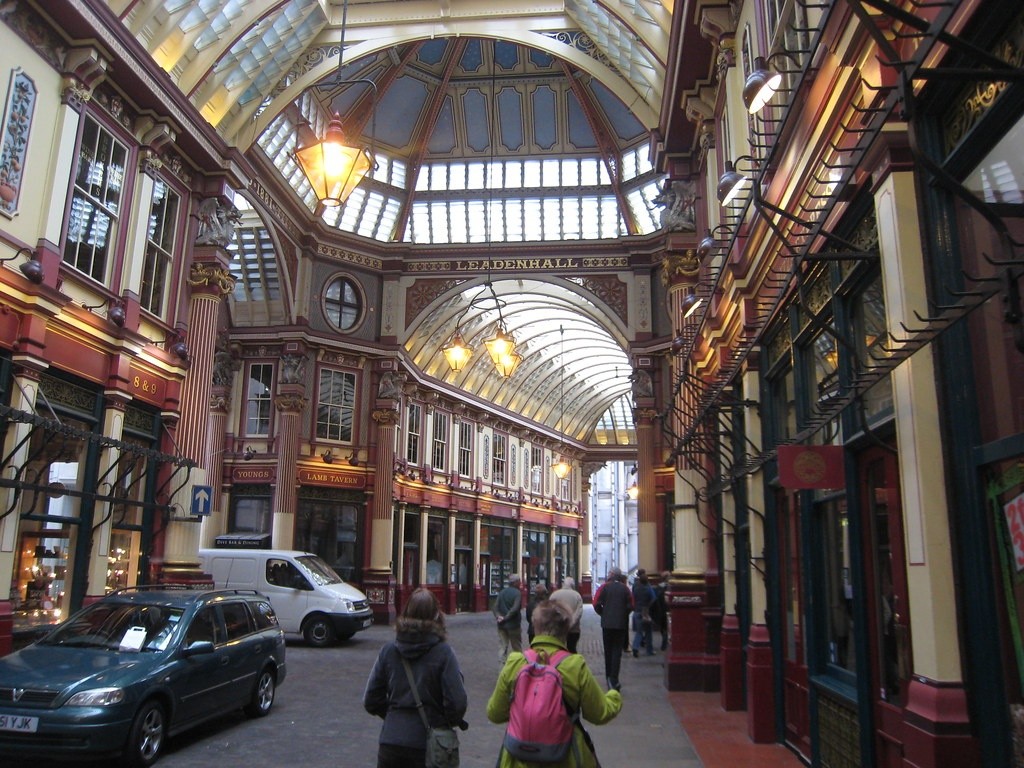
[661,642,669,651]
[624,647,632,653]
[647,650,657,655]
[632,648,639,658]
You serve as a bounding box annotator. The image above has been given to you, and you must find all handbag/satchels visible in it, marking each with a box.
[424,727,460,768]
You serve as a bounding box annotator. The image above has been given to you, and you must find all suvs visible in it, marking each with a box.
[0,584,288,767]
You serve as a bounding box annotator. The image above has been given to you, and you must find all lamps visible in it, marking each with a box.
[696,225,735,265]
[291,0,381,203]
[0,246,45,283]
[444,474,456,489]
[672,326,699,353]
[410,469,416,481]
[717,155,765,207]
[464,483,480,495]
[321,449,334,465]
[439,40,526,382]
[151,328,190,361]
[552,325,570,479]
[83,293,126,328]
[494,490,587,515]
[742,51,808,116]
[631,459,638,475]
[345,446,360,466]
[398,463,408,475]
[243,443,257,461]
[628,479,638,499]
[681,281,712,319]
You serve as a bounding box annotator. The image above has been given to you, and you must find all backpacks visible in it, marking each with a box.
[504,650,574,764]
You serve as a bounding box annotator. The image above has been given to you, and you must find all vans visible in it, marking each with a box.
[198,549,375,648]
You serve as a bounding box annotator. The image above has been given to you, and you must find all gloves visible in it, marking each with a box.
[606,677,621,693]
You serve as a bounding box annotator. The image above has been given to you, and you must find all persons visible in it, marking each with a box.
[593,567,635,692]
[621,568,671,658]
[493,574,583,677]
[487,600,622,768]
[362,587,467,768]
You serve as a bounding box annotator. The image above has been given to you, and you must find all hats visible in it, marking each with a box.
[610,567,621,577]
[507,573,520,583]
